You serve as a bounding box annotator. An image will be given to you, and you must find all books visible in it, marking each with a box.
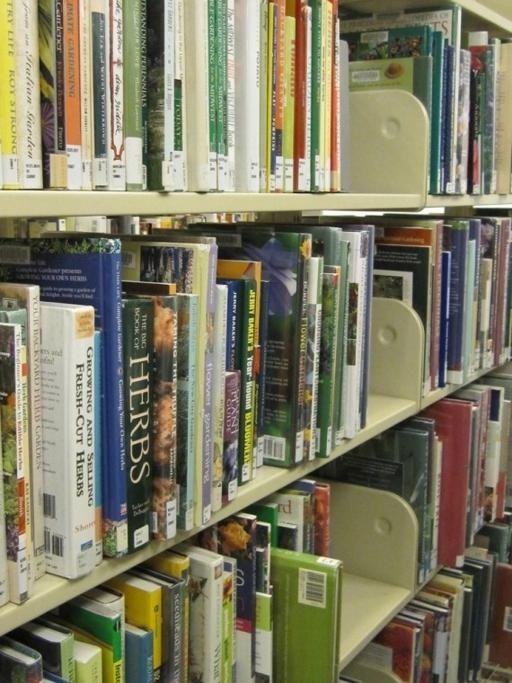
[351,11,500,197]
[371,209,512,393]
[0,479,342,681]
[0,213,367,608]
[311,373,511,583]
[1,0,344,192]
[340,555,512,681]
